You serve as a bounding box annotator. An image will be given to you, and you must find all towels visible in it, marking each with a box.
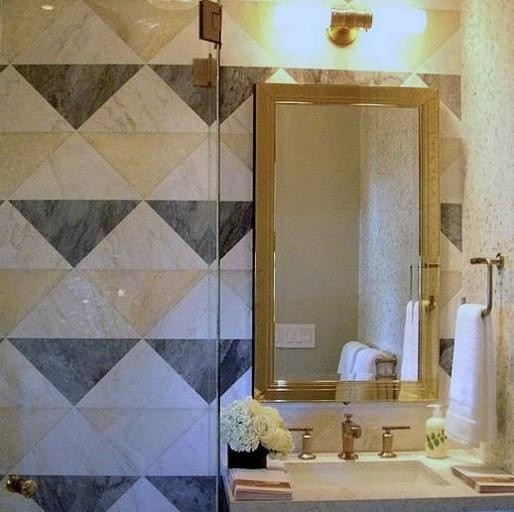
[400,301,418,380]
[444,303,497,447]
[337,340,393,382]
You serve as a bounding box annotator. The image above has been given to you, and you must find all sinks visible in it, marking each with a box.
[283,460,450,492]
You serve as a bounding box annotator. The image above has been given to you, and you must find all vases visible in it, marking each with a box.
[228,439,268,471]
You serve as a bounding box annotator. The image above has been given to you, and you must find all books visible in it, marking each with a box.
[228,468,293,501]
[451,463,514,493]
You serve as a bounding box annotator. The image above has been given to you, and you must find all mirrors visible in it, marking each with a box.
[252,83,442,402]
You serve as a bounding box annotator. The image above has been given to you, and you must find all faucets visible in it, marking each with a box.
[341,412,362,453]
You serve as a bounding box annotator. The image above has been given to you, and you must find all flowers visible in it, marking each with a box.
[219,395,297,461]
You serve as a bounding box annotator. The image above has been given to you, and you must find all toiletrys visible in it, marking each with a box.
[426,404,447,458]
